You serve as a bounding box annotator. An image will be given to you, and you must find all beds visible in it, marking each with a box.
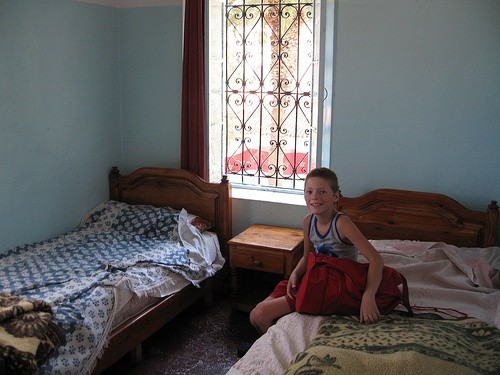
[225,189,500,375]
[0,166,231,375]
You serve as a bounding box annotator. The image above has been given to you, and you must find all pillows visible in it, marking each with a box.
[80,200,214,239]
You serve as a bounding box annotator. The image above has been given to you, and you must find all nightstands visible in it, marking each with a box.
[227,223,305,319]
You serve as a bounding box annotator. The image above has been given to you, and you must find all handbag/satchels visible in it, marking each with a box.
[295,254,415,320]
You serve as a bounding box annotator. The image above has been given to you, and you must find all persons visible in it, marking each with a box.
[250,167,384,334]
